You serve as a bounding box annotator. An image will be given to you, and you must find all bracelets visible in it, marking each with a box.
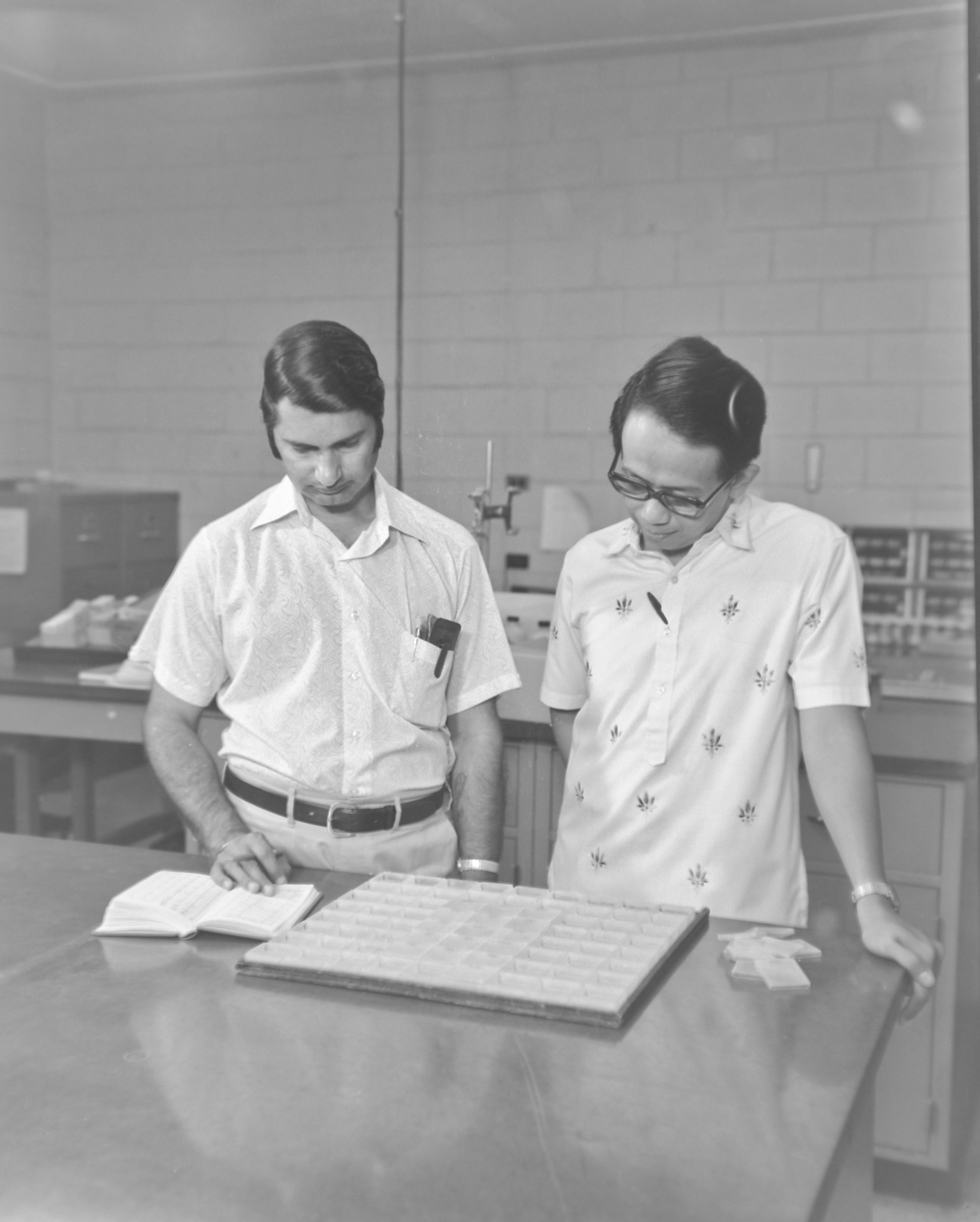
[221,842,229,849]
[456,859,499,875]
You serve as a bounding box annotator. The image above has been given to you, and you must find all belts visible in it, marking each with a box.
[221,766,443,833]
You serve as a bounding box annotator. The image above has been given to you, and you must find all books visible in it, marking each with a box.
[91,873,325,942]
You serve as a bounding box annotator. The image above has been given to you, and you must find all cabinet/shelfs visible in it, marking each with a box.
[504,746,980,1171]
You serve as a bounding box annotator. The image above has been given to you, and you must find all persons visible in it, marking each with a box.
[539,334,943,1025]
[128,318,521,898]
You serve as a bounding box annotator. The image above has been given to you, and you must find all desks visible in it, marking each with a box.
[0,833,914,1222]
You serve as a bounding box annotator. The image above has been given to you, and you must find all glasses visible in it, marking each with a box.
[607,444,747,518]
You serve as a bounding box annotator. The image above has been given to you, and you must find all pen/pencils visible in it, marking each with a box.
[226,849,282,864]
[647,591,668,625]
[411,626,422,662]
[433,641,451,678]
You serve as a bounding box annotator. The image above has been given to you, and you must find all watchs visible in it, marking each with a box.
[852,882,901,915]
